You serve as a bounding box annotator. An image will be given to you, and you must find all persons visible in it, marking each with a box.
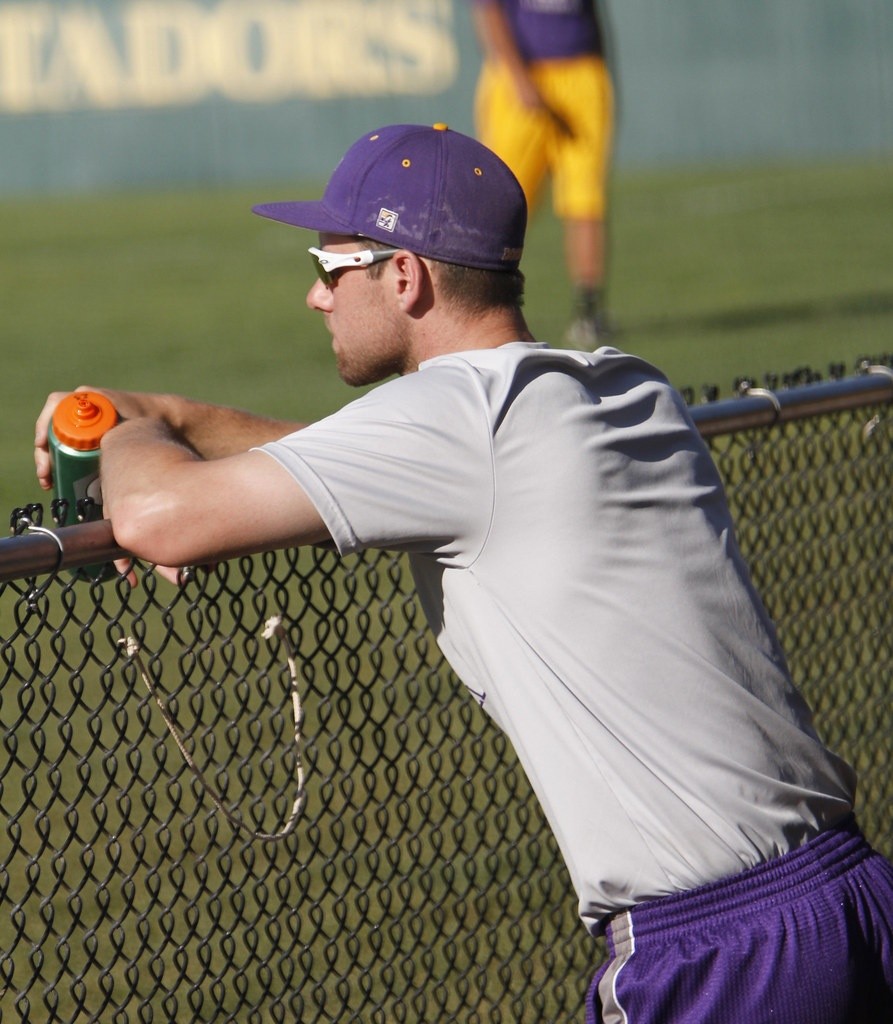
[470,0,618,352]
[35,123,893,1024]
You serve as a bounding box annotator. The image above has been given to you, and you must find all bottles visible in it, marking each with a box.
[47,393,118,580]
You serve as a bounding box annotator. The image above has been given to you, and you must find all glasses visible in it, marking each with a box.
[305,246,400,286]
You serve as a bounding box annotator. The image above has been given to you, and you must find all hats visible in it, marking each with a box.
[250,124,527,274]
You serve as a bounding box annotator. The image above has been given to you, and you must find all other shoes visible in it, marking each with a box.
[567,320,607,354]
